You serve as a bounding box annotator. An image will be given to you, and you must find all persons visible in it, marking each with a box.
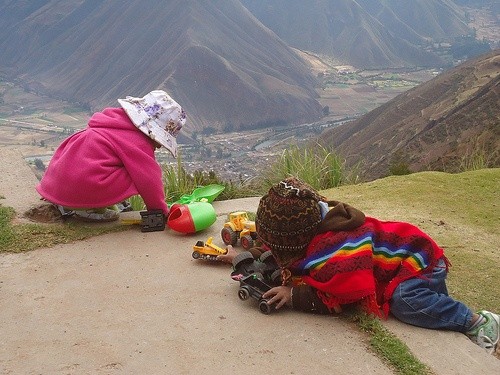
[217,177,500,355]
[35,90,187,222]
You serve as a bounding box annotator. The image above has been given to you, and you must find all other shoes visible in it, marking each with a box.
[76,209,120,221]
[54,202,75,216]
[464,310,500,355]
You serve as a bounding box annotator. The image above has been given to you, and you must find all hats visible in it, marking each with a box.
[255,177,329,269]
[117,89,188,159]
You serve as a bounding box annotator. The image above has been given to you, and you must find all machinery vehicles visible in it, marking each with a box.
[191,236,234,265]
[238,274,287,314]
[220,211,259,250]
[231,250,284,284]
